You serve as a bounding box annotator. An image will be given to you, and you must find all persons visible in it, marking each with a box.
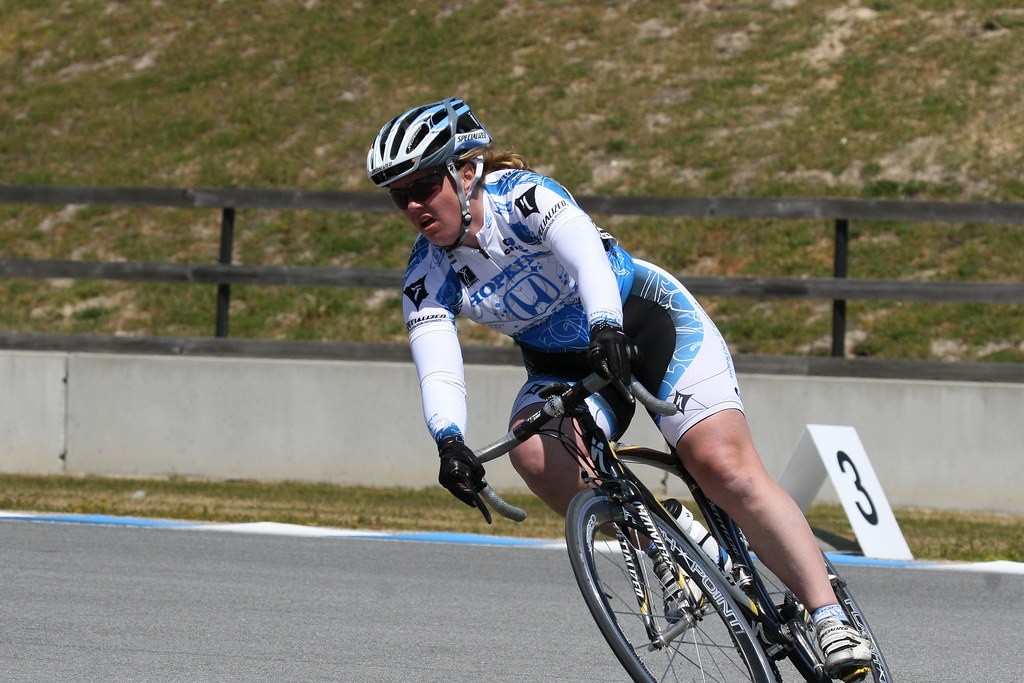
[365,98,873,683]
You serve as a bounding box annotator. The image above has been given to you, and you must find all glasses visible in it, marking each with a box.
[388,160,477,213]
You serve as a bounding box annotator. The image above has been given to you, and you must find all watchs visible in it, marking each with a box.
[591,320,622,339]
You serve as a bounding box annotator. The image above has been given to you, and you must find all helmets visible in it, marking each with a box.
[366,96,494,189]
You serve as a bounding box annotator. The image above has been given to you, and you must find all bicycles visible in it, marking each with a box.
[446,348,892,683]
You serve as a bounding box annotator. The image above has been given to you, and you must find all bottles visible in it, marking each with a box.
[660,498,732,572]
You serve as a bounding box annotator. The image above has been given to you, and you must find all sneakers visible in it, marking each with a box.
[645,542,691,623]
[816,616,872,678]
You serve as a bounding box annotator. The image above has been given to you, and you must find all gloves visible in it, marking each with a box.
[586,320,639,386]
[439,435,487,508]
